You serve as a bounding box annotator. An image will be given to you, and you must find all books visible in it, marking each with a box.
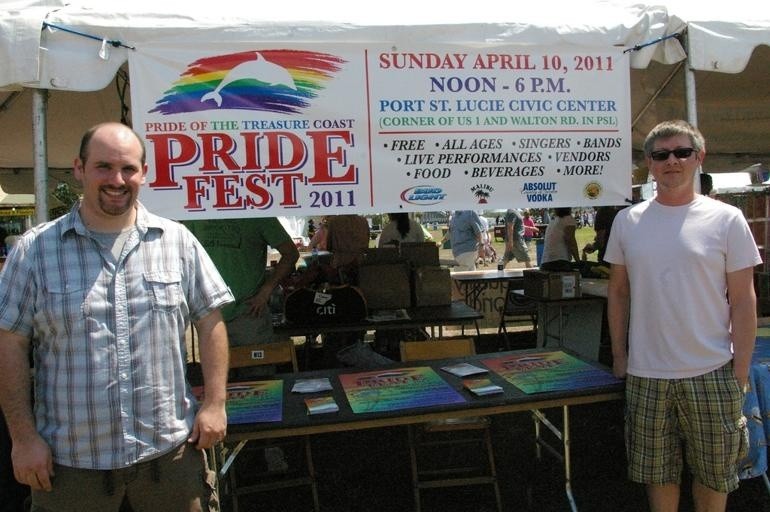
[305,397,338,415]
[442,363,489,377]
[463,378,503,396]
[292,378,332,394]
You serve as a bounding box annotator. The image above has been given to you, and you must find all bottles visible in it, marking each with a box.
[268,260,285,326]
[497,257,505,277]
[311,246,319,265]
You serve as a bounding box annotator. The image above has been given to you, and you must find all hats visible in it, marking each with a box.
[522,207,531,214]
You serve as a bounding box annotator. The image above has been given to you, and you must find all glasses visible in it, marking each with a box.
[650,147,697,162]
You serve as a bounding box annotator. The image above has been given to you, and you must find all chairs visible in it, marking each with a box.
[220,341,321,510]
[396,338,502,512]
[498,279,539,335]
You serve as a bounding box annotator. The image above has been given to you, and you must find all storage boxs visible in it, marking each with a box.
[400,241,442,266]
[359,262,409,310]
[523,269,552,299]
[550,270,584,298]
[412,266,452,306]
[359,247,398,265]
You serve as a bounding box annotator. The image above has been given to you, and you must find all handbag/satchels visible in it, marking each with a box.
[283,283,368,328]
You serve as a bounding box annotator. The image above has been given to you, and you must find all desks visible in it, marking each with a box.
[511,290,599,350]
[270,301,485,342]
[186,347,629,512]
[450,267,541,338]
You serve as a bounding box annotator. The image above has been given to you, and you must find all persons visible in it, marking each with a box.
[436,211,484,271]
[604,120,764,511]
[0,124,233,512]
[583,211,591,227]
[536,209,550,267]
[377,213,425,248]
[699,172,712,199]
[496,216,499,225]
[523,212,534,247]
[318,215,369,264]
[497,209,534,269]
[427,220,430,231]
[308,219,317,250]
[541,208,581,271]
[583,206,621,266]
[575,211,582,229]
[180,218,299,378]
